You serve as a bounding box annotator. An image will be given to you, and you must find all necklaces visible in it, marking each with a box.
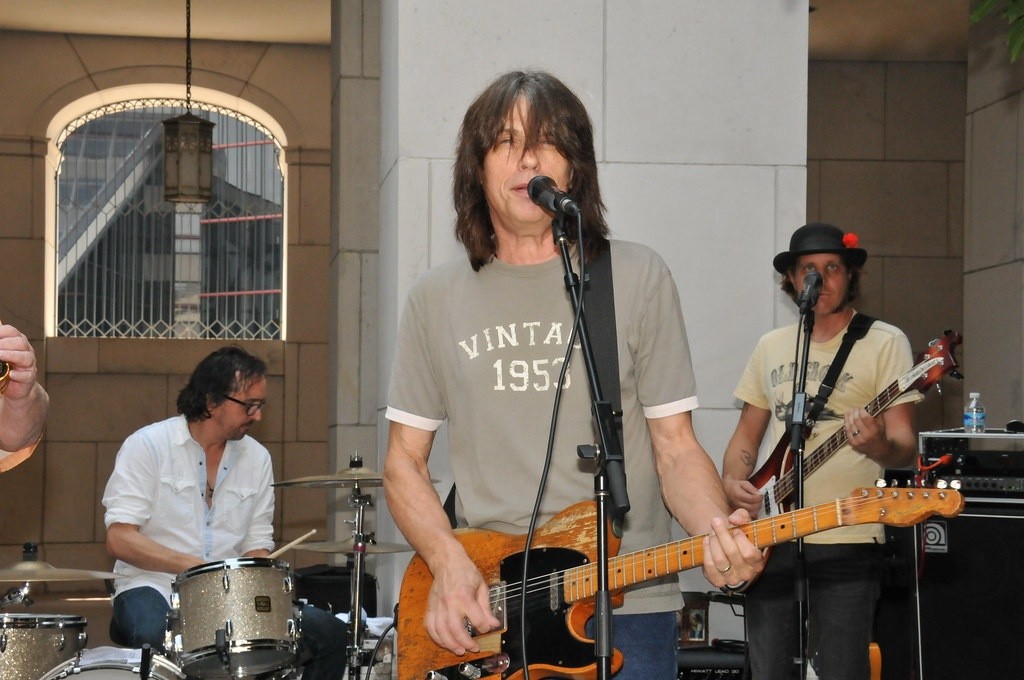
[188,425,214,497]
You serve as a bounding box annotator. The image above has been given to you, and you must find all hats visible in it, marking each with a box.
[774,223,867,276]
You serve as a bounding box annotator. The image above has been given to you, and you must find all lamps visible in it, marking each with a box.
[159,0,214,204]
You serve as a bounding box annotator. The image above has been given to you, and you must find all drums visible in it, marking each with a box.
[41,647,187,680]
[172,557,303,680]
[0,612,85,680]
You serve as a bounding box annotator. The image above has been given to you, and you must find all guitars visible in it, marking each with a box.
[397,477,965,680]
[703,329,963,595]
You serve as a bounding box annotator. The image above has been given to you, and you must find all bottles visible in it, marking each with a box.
[963,393,985,434]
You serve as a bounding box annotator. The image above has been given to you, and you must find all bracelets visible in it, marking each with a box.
[725,582,747,591]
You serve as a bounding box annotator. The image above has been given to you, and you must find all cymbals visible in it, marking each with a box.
[291,537,416,560]
[0,563,126,583]
[270,470,441,490]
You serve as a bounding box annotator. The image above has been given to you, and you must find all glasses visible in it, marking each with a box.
[223,394,265,416]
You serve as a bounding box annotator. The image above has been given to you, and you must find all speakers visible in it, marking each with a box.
[873,496,1024,680]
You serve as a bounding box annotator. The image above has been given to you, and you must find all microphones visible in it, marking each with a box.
[527,175,583,217]
[799,271,822,314]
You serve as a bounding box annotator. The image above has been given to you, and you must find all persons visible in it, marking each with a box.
[383,73,765,680]
[721,224,925,680]
[0,320,49,473]
[101,347,347,680]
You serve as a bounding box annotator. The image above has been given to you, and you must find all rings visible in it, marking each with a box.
[721,566,730,573]
[853,431,859,436]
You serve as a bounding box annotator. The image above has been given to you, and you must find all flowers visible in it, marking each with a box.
[843,232,860,247]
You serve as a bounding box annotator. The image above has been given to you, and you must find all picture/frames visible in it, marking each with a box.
[678,591,709,649]
